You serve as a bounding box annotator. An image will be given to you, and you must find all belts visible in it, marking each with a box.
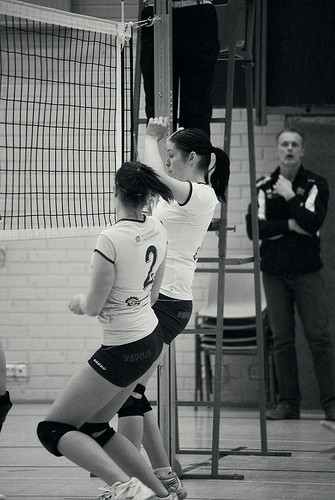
[145,0,212,8]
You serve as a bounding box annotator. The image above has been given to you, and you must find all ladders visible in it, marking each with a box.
[163,0,293,480]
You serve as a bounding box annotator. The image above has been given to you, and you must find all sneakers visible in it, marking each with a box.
[97,477,155,499]
[266,401,300,419]
[325,408,335,420]
[155,492,178,500]
[155,472,187,499]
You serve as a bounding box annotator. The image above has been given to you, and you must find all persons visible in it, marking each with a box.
[245,128,335,420]
[0,343,14,432]
[37,162,177,500]
[118,117,231,500]
[140,0,229,136]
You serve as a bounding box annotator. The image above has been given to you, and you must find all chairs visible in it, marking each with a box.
[194,254,276,413]
